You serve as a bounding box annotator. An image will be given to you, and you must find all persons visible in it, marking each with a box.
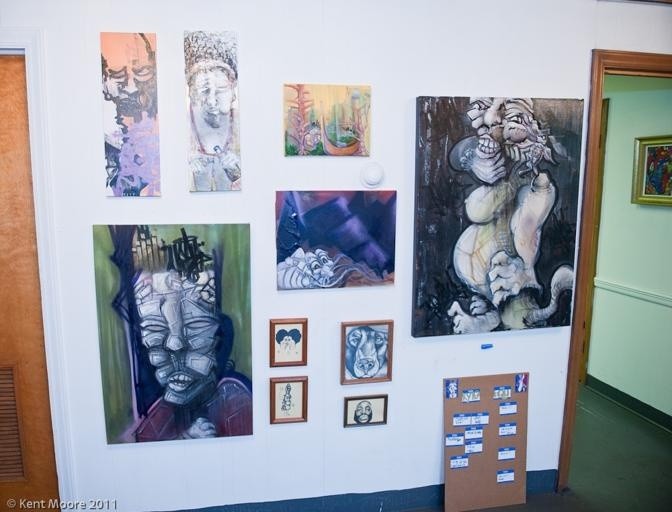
[184,31,240,190]
[101,32,160,196]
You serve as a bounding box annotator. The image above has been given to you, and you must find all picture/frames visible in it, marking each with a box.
[268,317,309,368]
[268,375,309,425]
[630,136,671,208]
[341,319,394,385]
[342,393,389,429]
